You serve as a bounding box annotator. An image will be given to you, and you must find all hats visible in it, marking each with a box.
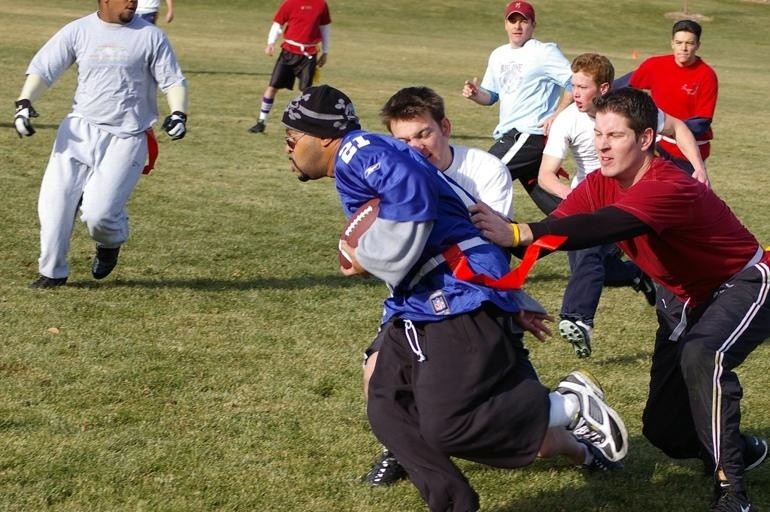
[504,0,535,22]
[280,84,361,139]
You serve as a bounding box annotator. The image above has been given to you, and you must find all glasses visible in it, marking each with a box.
[284,132,306,149]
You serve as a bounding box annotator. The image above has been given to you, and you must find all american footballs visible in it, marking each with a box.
[339,198,381,270]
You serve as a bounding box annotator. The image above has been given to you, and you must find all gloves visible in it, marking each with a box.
[163,111,187,141]
[13,99,39,139]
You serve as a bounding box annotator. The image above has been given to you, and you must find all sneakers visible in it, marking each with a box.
[360,450,409,488]
[249,119,266,133]
[553,367,629,463]
[558,319,592,358]
[27,276,68,289]
[631,270,656,306]
[92,243,119,279]
[741,434,768,471]
[568,439,625,481]
[711,492,753,512]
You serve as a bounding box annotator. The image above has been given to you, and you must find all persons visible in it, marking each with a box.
[628,19,719,187]
[466,86,769,512]
[12,1,188,290]
[245,1,332,134]
[135,1,174,29]
[536,53,712,360]
[357,85,516,489]
[280,84,631,510]
[461,1,575,218]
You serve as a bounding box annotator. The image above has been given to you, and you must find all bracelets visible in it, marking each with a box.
[511,223,520,248]
